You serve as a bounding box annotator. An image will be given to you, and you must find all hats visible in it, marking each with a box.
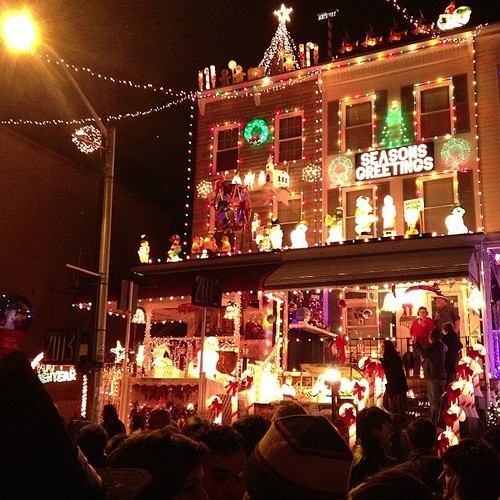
[239,413,352,500]
[434,296,448,303]
[270,400,308,424]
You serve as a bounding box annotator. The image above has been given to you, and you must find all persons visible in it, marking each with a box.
[409,295,463,428]
[379,340,410,413]
[67,401,500,500]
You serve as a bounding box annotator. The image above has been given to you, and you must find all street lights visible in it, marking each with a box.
[1,0,116,422]
